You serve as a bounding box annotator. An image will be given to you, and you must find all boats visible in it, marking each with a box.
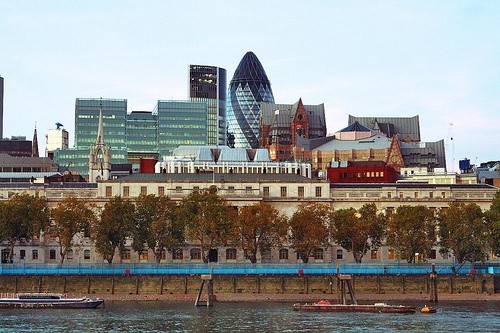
[293,301,416,313]
[0,292,104,309]
